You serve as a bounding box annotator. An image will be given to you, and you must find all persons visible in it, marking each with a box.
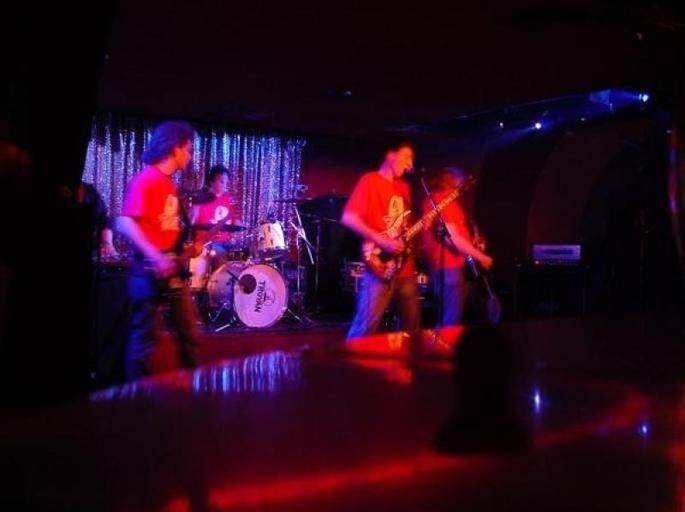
[79,182,116,257]
[422,167,494,327]
[340,143,432,337]
[189,244,212,288]
[120,122,205,382]
[187,166,243,269]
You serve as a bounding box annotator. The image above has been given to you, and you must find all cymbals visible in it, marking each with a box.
[272,197,314,202]
[192,224,249,232]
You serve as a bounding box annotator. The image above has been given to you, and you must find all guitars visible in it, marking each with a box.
[360,174,479,281]
[468,215,487,253]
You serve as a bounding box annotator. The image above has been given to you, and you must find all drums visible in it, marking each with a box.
[246,220,289,262]
[183,241,212,292]
[205,260,290,327]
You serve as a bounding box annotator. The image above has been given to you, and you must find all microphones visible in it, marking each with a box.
[405,167,435,177]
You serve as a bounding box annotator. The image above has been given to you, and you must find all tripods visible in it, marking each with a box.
[279,205,344,329]
